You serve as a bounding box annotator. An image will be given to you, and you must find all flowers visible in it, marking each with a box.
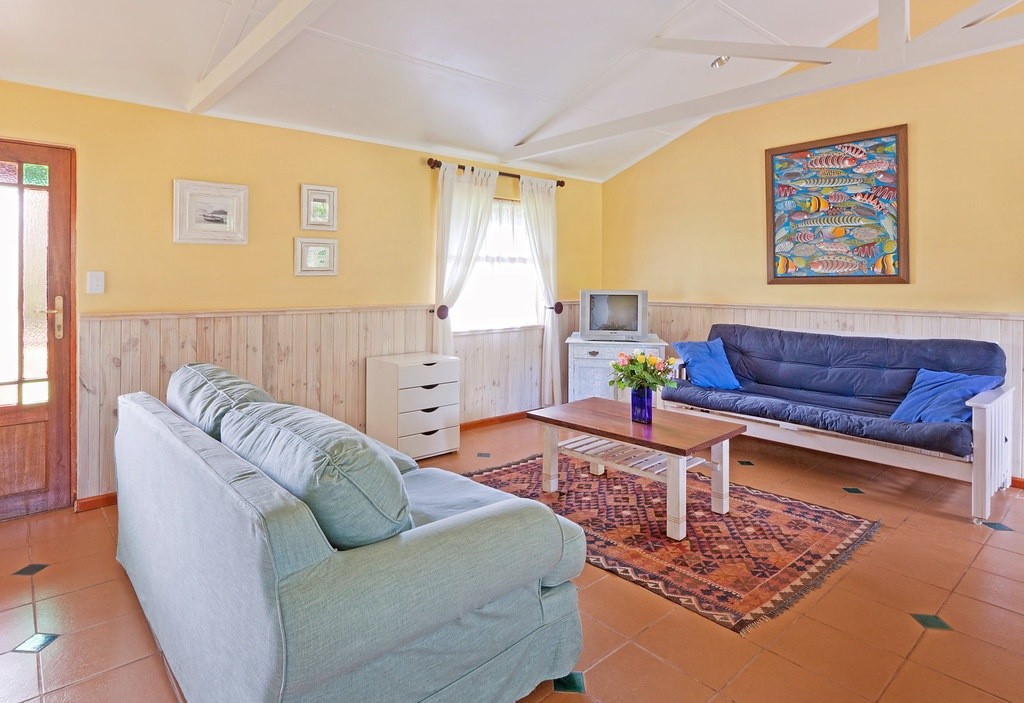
[606,348,678,393]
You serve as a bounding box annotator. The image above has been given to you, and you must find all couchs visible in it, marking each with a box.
[113,391,587,703]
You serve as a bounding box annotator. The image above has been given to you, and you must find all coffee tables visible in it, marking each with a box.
[526,396,747,542]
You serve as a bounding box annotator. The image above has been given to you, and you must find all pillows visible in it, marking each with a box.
[166,363,276,441]
[220,401,416,550]
[671,337,743,390]
[889,368,1005,422]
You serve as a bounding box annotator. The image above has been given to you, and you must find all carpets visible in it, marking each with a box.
[461,450,884,641]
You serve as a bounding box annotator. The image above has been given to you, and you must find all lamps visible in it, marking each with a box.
[710,55,729,68]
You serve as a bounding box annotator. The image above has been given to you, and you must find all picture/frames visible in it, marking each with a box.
[764,123,910,285]
[300,183,338,231]
[172,178,249,244]
[293,237,339,275]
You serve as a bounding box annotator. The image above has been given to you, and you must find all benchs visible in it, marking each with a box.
[659,323,1016,526]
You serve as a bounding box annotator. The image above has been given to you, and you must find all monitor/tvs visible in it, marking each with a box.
[580,290,649,342]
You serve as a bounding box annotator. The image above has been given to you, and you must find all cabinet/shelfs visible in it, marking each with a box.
[564,331,670,403]
[365,352,462,461]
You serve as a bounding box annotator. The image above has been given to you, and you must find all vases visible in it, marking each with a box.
[630,385,652,424]
